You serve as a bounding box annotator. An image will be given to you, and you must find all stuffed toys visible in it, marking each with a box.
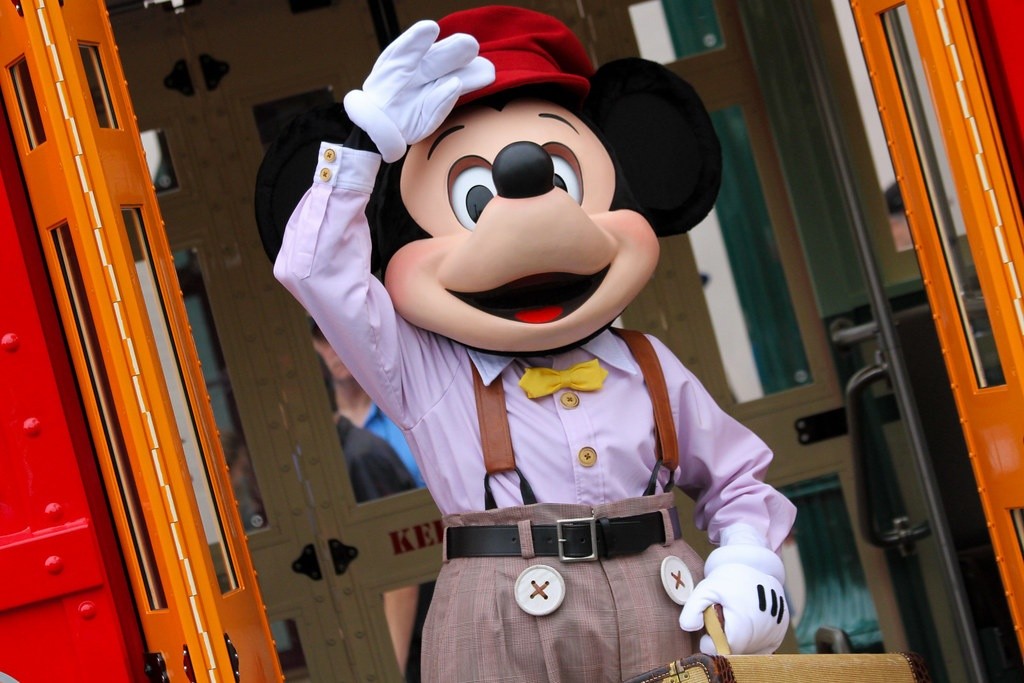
[248,5,802,683]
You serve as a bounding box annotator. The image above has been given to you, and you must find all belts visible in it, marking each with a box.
[445,505,681,563]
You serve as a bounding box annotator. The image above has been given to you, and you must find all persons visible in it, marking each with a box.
[224,308,445,683]
